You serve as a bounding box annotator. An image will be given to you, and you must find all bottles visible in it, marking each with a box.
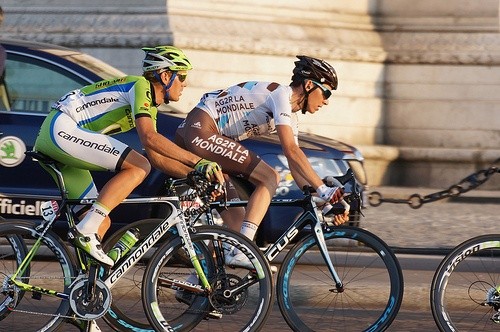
[106,227,141,264]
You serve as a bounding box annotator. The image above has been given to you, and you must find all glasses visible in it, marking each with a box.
[166,71,187,82]
[305,79,332,99]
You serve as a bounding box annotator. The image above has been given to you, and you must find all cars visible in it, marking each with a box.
[1,38,368,266]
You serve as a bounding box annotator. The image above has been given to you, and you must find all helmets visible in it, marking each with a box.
[142,46,192,71]
[293,55,338,90]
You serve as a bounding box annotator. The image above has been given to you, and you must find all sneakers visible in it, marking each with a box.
[64,311,102,332]
[226,248,277,272]
[221,242,232,256]
[66,225,114,266]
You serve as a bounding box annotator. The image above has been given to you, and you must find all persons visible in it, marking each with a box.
[173,54,350,318]
[30,44,226,332]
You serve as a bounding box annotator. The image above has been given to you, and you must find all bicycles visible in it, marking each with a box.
[429,233,500,332]
[0,149,274,332]
[96,167,405,332]
